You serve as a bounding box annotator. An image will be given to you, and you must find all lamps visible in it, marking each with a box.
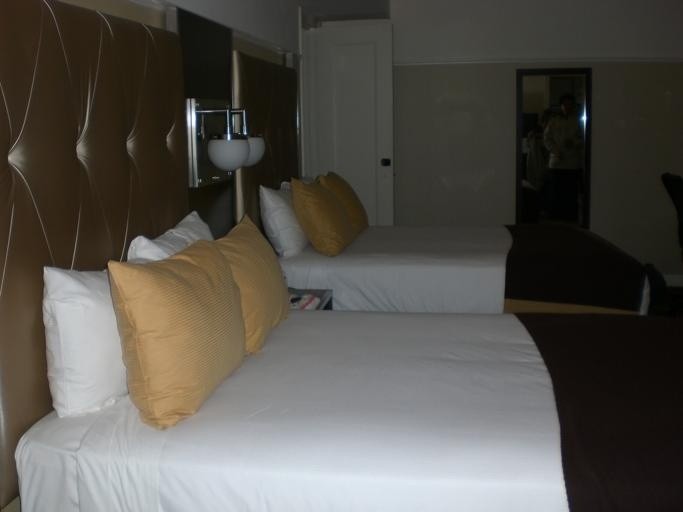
[185,96,269,190]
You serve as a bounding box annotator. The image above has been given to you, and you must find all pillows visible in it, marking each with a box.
[213,213,289,356]
[255,171,367,262]
[127,211,215,264]
[107,239,246,429]
[43,266,129,419]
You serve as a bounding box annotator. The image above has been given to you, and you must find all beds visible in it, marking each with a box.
[270,221,664,316]
[13,309,683,512]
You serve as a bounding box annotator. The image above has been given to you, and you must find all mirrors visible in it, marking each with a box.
[512,65,593,229]
